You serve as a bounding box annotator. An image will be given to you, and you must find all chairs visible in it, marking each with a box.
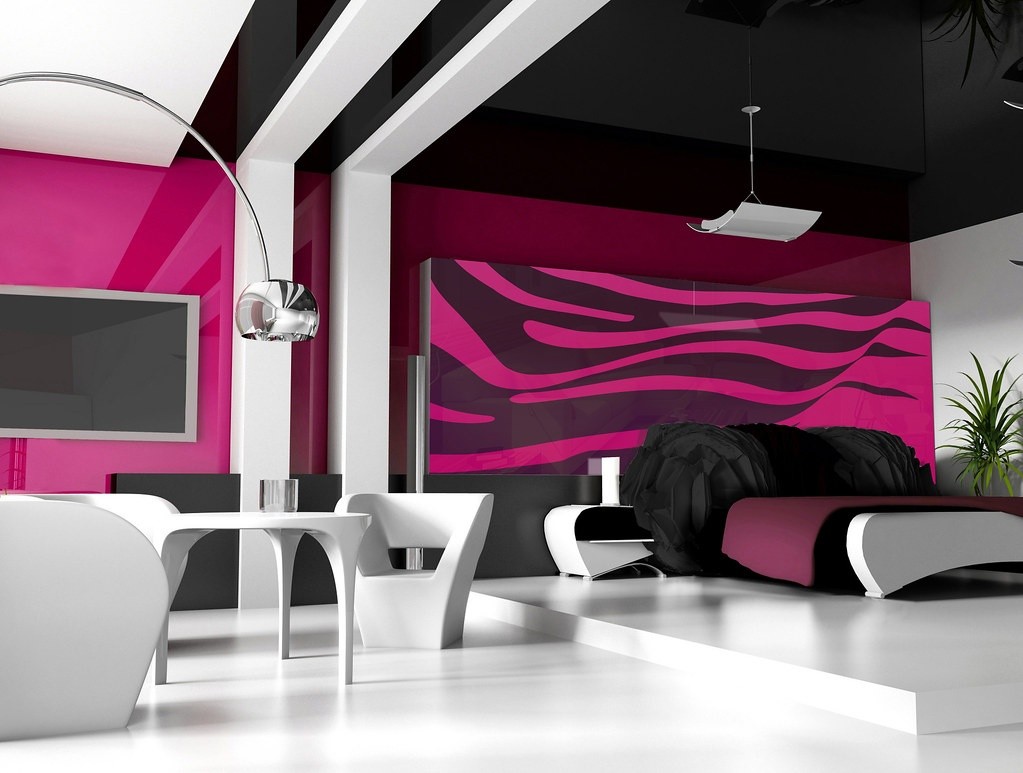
[0,481,194,740]
[332,492,494,650]
[543,504,668,584]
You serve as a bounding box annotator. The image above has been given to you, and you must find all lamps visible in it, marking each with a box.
[687,106,822,240]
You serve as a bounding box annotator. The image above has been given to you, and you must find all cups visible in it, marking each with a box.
[260,479,299,512]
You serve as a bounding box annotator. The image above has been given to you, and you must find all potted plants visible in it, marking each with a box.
[934,349,1023,500]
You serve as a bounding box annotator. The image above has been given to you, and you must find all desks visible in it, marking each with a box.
[721,493,1023,600]
[149,506,373,685]
